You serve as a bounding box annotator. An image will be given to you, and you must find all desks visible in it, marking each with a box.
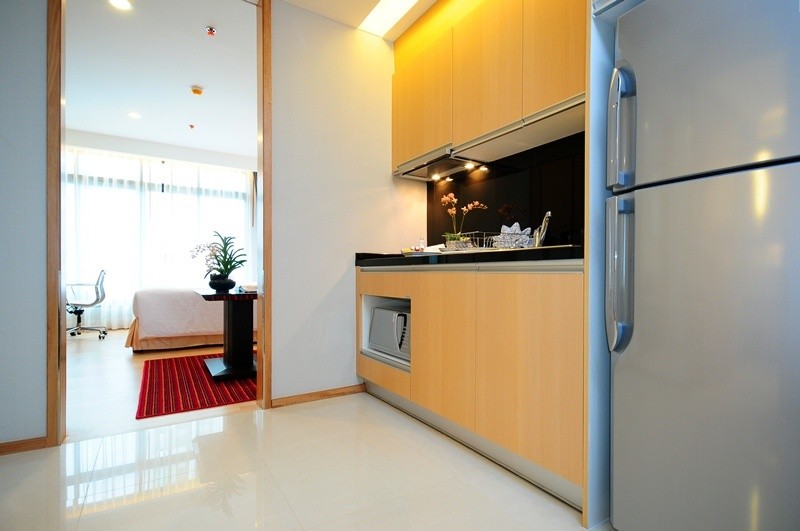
[193,290,257,380]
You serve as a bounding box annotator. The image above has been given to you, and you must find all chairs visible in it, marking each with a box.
[65,269,109,339]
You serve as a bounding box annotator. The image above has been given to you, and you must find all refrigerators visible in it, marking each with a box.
[605,1,799,530]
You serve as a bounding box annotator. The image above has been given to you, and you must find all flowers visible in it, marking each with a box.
[190,231,247,280]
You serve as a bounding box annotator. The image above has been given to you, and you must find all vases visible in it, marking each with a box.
[209,274,236,292]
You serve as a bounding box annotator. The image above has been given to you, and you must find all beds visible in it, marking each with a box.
[125,288,258,352]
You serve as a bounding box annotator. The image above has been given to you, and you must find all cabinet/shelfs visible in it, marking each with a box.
[390,1,586,174]
[409,271,585,491]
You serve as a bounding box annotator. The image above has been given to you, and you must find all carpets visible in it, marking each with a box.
[134,349,258,420]
